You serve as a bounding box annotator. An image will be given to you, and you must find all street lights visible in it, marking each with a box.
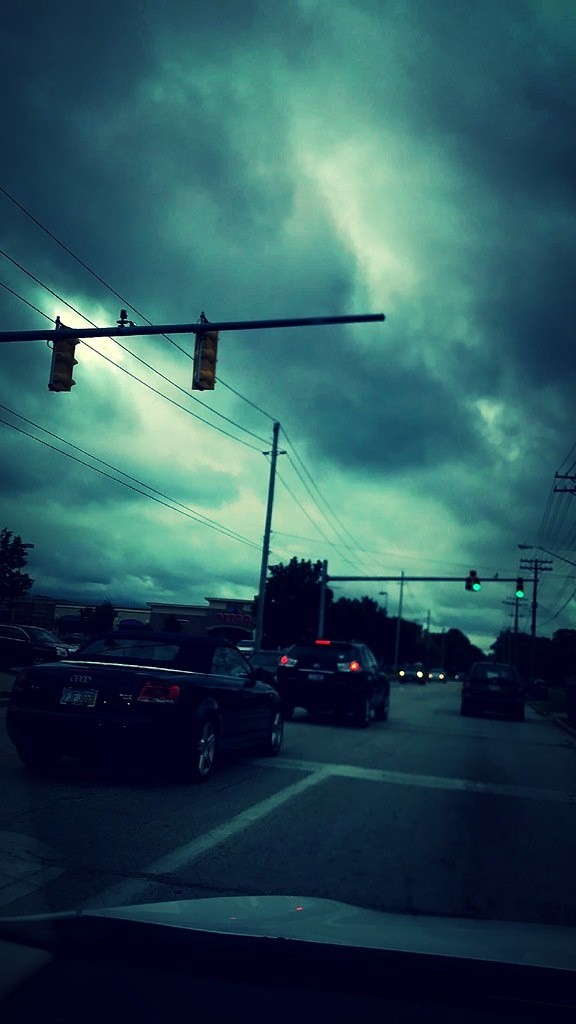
[380,590,389,638]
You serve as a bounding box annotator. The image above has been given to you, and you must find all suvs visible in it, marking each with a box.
[276,637,390,729]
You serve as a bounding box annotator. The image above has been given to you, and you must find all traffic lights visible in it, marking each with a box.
[472,578,480,592]
[465,577,471,590]
[515,577,525,598]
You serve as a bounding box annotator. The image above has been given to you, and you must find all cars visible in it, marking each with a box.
[428,669,447,683]
[6,627,285,783]
[63,633,87,646]
[58,614,86,633]
[116,618,154,632]
[0,622,77,667]
[460,661,526,722]
[398,664,426,685]
